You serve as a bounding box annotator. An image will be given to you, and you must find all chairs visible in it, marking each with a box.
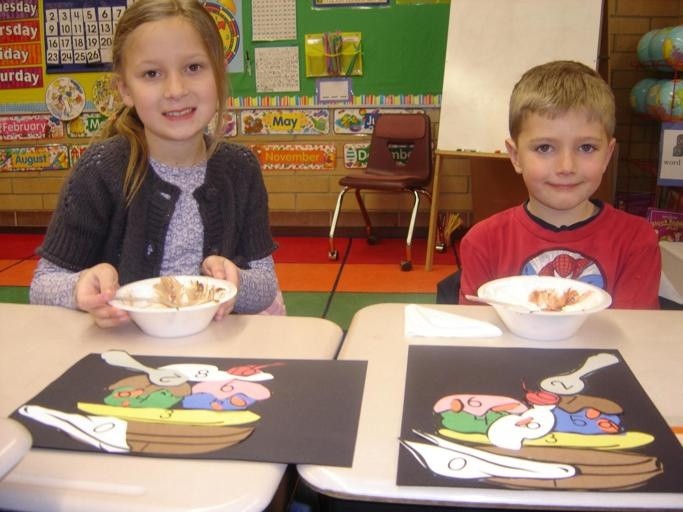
[328,113,447,271]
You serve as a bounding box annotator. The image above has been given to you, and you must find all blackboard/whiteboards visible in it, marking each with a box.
[435,0,602,158]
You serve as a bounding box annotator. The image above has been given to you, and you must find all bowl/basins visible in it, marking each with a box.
[477,274,612,340]
[109,275,238,339]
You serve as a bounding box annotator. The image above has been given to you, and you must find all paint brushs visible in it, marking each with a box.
[439,211,464,248]
[324,32,341,74]
[346,41,361,75]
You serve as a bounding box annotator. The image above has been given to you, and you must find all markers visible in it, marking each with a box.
[456,149,476,152]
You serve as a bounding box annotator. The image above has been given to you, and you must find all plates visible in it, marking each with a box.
[93,74,124,117]
[46,78,86,121]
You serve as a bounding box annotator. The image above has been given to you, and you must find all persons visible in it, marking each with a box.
[27,1,281,331]
[459,60,661,310]
